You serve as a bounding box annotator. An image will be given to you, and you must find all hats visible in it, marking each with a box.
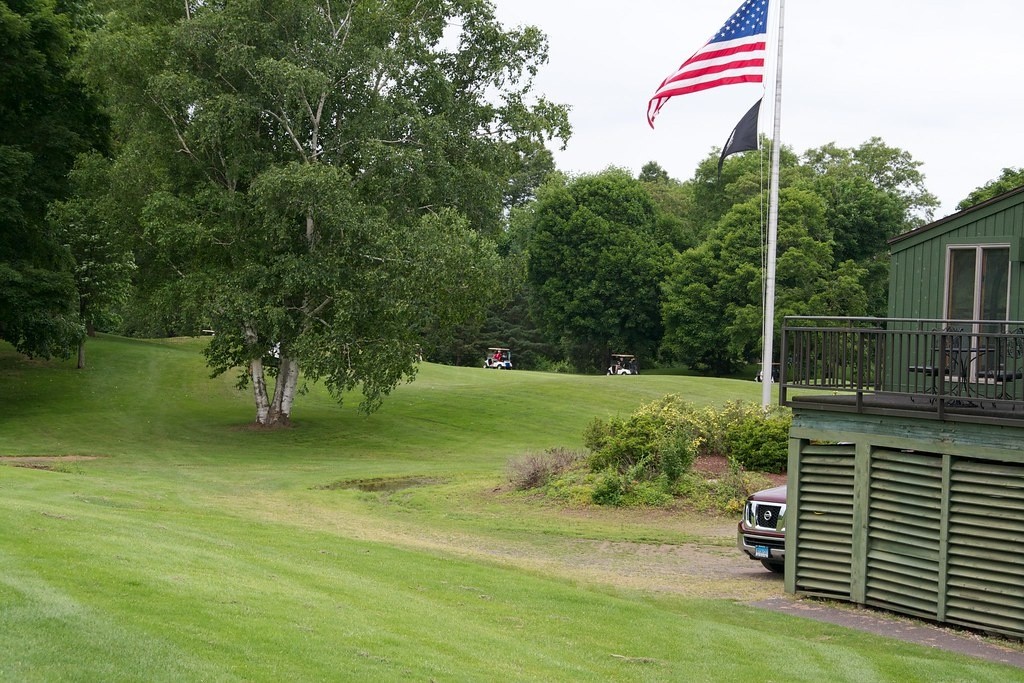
[498,351,501,353]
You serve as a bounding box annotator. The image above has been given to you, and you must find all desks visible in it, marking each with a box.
[931,347,995,407]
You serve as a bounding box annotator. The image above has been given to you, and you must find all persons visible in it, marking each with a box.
[493,350,507,362]
[620,357,629,369]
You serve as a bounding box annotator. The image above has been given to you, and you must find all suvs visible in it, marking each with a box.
[737,485,787,574]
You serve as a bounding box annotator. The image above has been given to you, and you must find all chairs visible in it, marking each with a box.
[975,328,1024,411]
[909,326,963,403]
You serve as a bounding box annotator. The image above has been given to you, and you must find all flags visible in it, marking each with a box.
[717,95,761,186]
[647,0,769,129]
